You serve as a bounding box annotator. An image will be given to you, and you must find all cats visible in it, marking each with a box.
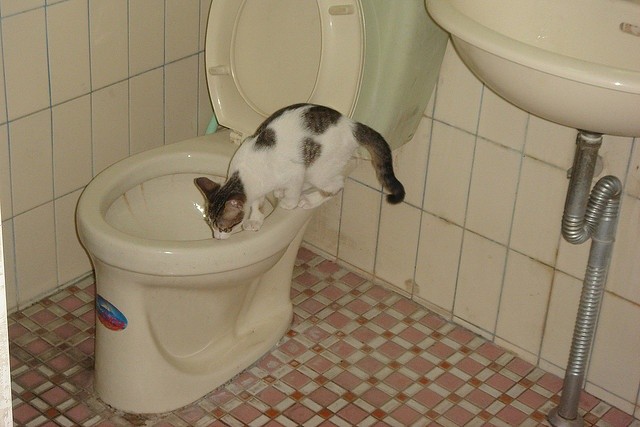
[192,103,405,240]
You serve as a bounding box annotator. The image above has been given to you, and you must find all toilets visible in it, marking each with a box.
[75,0,449,417]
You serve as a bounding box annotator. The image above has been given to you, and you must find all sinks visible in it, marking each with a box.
[424,0,640,138]
